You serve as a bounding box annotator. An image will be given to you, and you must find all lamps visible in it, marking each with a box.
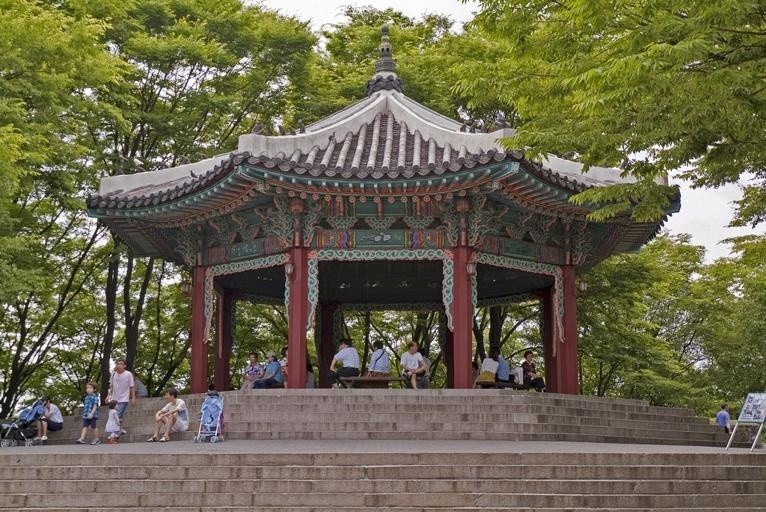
[572,280,590,299]
[178,281,192,296]
[283,260,296,281]
[464,260,476,280]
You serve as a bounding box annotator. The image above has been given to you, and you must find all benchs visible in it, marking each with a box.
[266,384,284,388]
[476,380,529,389]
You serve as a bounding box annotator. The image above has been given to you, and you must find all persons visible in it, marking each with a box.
[715,402,733,435]
[400,341,430,389]
[107,358,136,433]
[327,338,390,389]
[32,396,64,442]
[472,346,546,392]
[132,372,148,398]
[104,399,121,444]
[241,347,315,389]
[73,381,101,445]
[146,389,189,443]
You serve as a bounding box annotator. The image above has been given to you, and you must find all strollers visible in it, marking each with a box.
[190,392,226,443]
[0,394,51,448]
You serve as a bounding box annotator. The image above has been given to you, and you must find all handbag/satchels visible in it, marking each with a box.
[106,389,112,404]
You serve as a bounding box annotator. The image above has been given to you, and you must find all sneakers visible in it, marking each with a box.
[77,437,103,446]
[34,435,48,441]
[145,433,170,442]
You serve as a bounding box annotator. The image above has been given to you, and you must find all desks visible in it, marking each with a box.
[339,374,421,388]
